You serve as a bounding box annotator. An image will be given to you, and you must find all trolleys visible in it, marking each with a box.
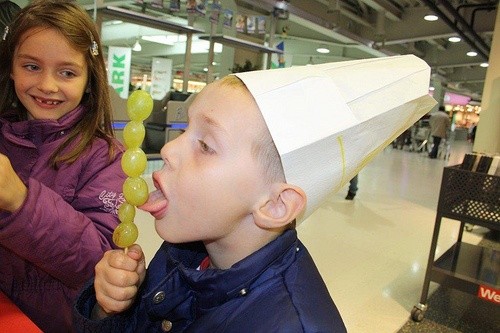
[409,122,432,153]
[437,132,452,161]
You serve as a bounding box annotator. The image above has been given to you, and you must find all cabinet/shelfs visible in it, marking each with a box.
[411,164,500,323]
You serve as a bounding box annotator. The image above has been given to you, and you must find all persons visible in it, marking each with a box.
[345,175,359,200]
[73,54,438,333]
[0,0,129,333]
[393,105,476,161]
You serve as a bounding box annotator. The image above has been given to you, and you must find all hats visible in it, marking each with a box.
[228,54,439,229]
